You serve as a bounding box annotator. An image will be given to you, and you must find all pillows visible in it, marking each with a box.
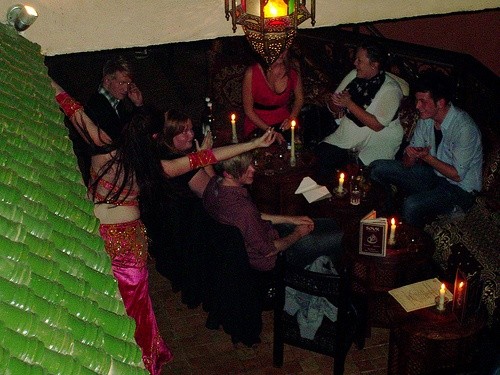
[473,115,500,209]
[329,92,420,139]
[206,36,327,113]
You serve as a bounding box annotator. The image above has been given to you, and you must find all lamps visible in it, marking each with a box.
[7,4,38,31]
[223,0,316,71]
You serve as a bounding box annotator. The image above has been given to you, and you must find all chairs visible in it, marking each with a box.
[146,174,201,309]
[192,198,262,346]
[272,251,371,375]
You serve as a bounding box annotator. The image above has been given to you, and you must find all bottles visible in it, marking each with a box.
[200,97,218,146]
[335,149,372,200]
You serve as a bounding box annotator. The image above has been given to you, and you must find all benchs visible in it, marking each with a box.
[41,29,500,323]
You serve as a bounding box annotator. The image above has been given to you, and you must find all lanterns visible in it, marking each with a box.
[225,0,315,67]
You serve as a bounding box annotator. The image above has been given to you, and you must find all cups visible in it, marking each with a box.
[351,187,361,206]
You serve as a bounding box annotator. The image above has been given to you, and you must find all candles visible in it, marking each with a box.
[290,120,296,158]
[230,114,238,143]
[457,282,464,305]
[389,218,396,244]
[438,282,446,310]
[339,172,345,192]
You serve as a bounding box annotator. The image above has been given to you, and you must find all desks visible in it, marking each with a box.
[342,219,437,329]
[305,176,402,263]
[386,280,489,375]
[251,141,320,217]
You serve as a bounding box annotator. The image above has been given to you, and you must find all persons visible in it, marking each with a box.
[202,143,315,270]
[317,43,410,175]
[152,107,217,198]
[51,80,278,375]
[367,80,482,228]
[241,48,305,143]
[87,55,143,139]
